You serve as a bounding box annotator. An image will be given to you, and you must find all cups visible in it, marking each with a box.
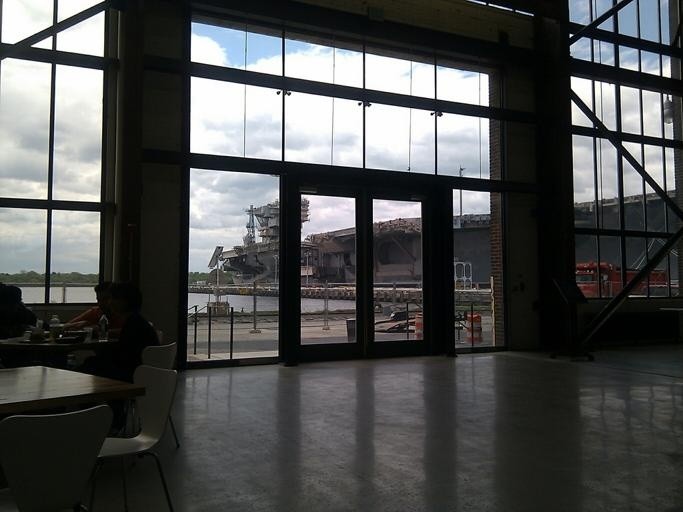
[84,327,93,338]
[36,319,43,328]
[50,324,64,339]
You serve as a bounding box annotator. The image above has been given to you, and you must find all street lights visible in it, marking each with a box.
[458,165,465,227]
[208,246,223,316]
[303,249,311,288]
[271,254,278,287]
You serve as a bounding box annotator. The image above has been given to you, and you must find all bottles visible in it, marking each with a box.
[98,314,109,342]
[49,314,59,343]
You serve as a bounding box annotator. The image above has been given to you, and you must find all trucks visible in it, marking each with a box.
[192,281,206,287]
[575,262,667,299]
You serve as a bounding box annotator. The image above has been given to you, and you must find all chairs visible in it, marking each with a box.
[0,404,114,511]
[123,335,180,470]
[82,365,178,512]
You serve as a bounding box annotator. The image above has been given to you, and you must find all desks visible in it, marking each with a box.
[0,365,145,489]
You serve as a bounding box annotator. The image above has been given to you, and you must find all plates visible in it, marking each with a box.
[18,330,90,343]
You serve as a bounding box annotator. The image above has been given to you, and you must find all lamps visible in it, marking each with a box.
[663,94,673,124]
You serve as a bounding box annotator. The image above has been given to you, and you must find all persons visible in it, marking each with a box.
[531,256,589,351]
[0,280,160,432]
